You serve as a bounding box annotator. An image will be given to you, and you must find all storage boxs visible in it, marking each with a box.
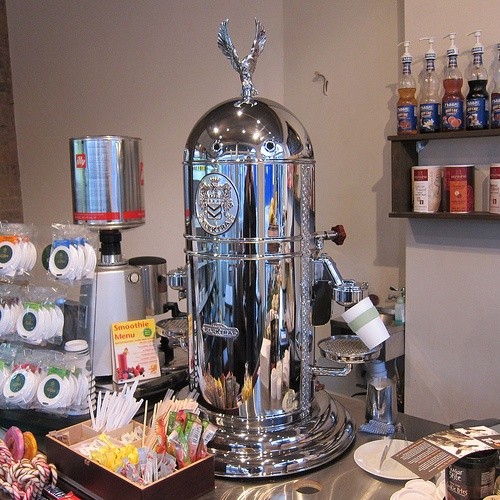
[47,415,217,500]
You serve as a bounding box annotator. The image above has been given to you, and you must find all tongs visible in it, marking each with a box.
[379,422,409,469]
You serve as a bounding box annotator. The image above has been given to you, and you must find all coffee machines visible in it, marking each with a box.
[68,135,146,382]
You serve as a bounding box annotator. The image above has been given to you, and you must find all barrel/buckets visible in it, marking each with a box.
[128,256,174,366]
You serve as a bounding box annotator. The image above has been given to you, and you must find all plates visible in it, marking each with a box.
[353,440,421,479]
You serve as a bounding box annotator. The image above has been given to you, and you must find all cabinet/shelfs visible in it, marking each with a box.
[388,128,500,219]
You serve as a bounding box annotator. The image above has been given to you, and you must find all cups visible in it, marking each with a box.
[340,297,391,351]
[411,165,441,213]
[63,339,97,416]
[442,165,476,213]
[487,163,500,214]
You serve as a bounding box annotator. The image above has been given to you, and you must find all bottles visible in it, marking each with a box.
[364,359,398,425]
[394,288,405,325]
